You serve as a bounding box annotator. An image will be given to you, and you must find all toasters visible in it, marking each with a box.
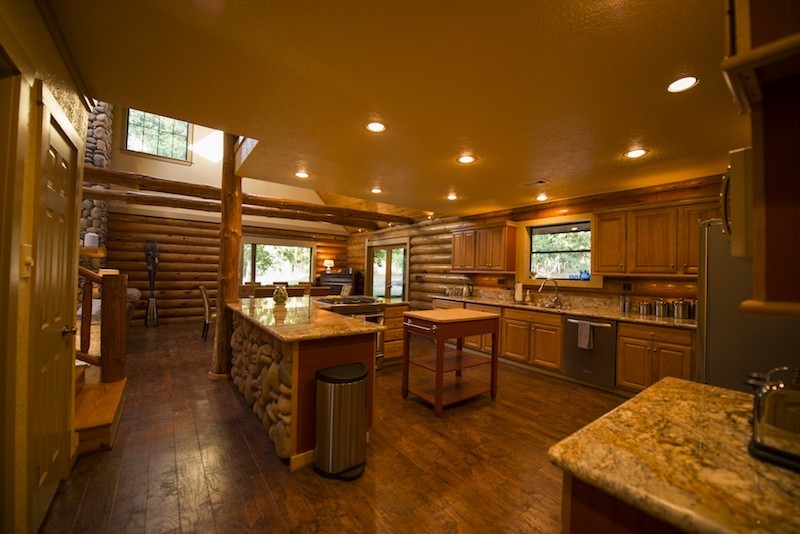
[745,365,800,459]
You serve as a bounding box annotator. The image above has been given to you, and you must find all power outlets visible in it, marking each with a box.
[498,280,505,284]
[622,283,633,292]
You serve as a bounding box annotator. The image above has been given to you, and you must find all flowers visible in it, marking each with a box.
[144,239,159,296]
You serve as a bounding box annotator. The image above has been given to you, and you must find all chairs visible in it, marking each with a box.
[286,289,305,297]
[310,287,331,296]
[245,282,261,294]
[274,282,289,294]
[199,285,217,341]
[340,285,352,296]
[300,282,313,294]
[255,288,275,298]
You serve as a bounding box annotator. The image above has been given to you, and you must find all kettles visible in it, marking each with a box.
[463,283,473,297]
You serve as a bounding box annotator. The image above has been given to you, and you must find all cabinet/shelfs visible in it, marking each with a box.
[501,308,563,376]
[616,322,695,395]
[464,303,501,358]
[432,299,464,350]
[382,306,411,369]
[450,225,475,273]
[591,211,626,276]
[475,221,517,274]
[319,271,364,295]
[628,198,731,278]
[401,308,502,418]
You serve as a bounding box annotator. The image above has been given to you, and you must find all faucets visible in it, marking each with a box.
[538,278,558,307]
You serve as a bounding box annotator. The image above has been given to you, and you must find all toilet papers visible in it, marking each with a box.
[514,283,523,301]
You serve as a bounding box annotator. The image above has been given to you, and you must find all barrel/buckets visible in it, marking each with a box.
[655,298,668,317]
[673,298,689,319]
[638,299,650,315]
[691,298,697,319]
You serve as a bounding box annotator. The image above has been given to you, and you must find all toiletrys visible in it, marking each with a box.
[524,289,532,304]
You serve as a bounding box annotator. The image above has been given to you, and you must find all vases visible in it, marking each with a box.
[145,298,158,327]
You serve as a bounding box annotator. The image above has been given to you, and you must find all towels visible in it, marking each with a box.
[577,320,593,350]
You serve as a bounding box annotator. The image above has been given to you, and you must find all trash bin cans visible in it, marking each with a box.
[313,362,369,480]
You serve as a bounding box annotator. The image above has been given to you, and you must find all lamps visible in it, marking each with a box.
[323,260,334,271]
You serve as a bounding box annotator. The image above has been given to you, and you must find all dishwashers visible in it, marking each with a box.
[559,314,617,390]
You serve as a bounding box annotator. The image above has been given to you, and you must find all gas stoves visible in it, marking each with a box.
[316,297,383,304]
